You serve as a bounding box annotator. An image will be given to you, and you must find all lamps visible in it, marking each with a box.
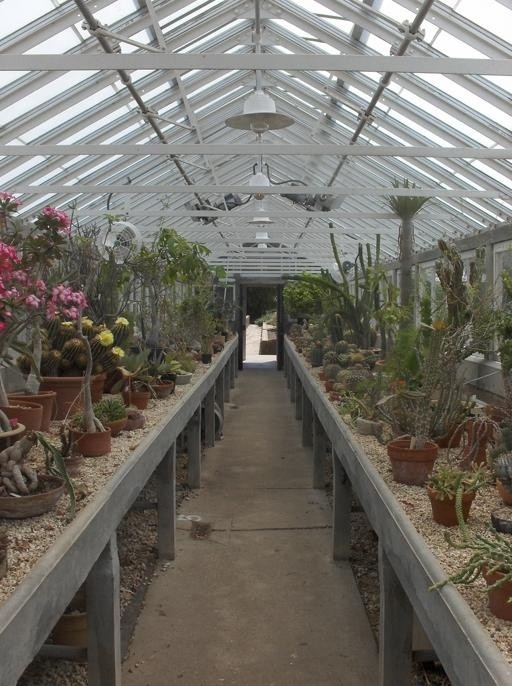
[242,149,278,257]
[225,0,297,143]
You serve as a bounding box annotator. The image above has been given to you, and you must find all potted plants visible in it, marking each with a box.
[424,525,512,622]
[50,590,88,647]
[285,178,386,440]
[1,429,66,520]
[425,462,494,527]
[492,503,512,534]
[386,317,448,487]
[1,188,237,458]
[448,178,511,502]
